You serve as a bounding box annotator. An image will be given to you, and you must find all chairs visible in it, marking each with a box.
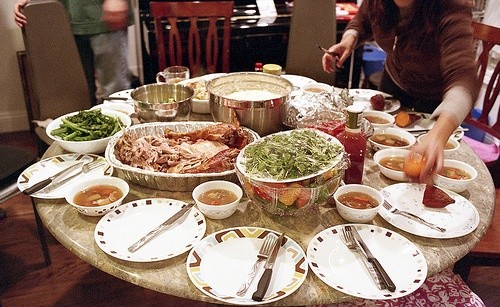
[148,1,232,83]
[457,20,500,284]
[0,0,95,263]
[286,0,336,87]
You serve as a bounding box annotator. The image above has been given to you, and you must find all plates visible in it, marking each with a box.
[185,226,308,305]
[17,153,114,200]
[378,182,481,239]
[307,224,428,300]
[90,88,135,115]
[93,198,206,263]
[393,112,465,140]
[339,88,401,114]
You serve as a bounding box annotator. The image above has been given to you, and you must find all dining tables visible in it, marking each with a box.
[35,113,495,307]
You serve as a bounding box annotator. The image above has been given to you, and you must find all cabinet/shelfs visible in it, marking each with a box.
[134,0,295,84]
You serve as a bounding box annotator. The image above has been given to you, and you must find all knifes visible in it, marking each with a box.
[127,199,196,253]
[22,162,84,195]
[350,225,396,292]
[251,231,286,301]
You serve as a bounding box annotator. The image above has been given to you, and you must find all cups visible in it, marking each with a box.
[155,65,190,84]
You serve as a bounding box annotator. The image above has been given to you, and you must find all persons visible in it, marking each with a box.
[322,0,480,184]
[14,0,136,104]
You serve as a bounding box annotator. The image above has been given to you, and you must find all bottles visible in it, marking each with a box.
[336,107,366,183]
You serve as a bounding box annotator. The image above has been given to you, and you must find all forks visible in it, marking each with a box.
[382,200,446,232]
[235,234,278,296]
[43,160,105,195]
[341,227,386,290]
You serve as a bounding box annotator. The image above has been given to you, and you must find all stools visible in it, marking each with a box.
[303,267,487,307]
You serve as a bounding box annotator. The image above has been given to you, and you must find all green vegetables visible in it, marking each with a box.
[49,108,127,142]
[239,128,343,181]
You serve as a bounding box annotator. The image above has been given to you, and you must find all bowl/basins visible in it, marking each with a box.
[333,184,383,224]
[431,159,478,193]
[46,109,132,154]
[368,126,416,150]
[362,110,395,128]
[417,133,461,158]
[104,71,345,219]
[372,148,427,182]
[64,176,130,217]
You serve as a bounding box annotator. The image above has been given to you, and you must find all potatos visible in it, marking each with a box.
[422,184,455,208]
[396,111,421,127]
[370,94,385,111]
